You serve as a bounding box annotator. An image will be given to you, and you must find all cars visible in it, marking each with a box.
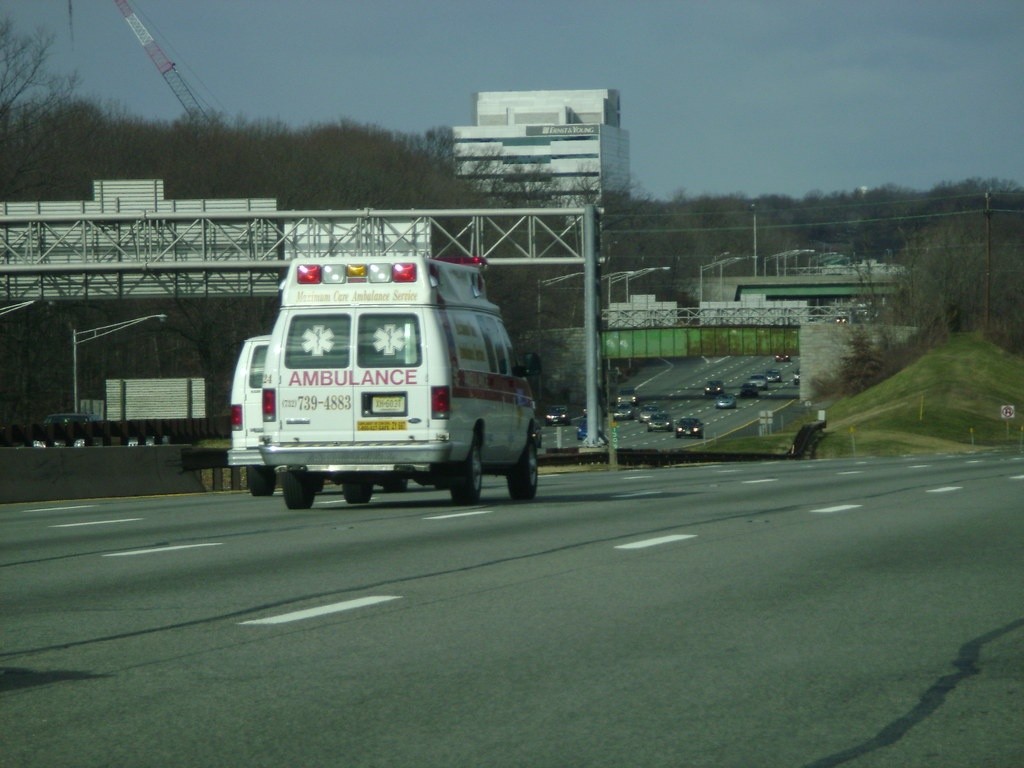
[647,410,673,432]
[766,368,783,383]
[546,404,571,428]
[748,374,769,390]
[792,367,800,385]
[675,418,706,439]
[614,402,634,421]
[704,380,724,395]
[835,316,849,324]
[639,404,660,424]
[775,353,791,361]
[576,418,587,441]
[716,392,738,408]
[739,382,759,397]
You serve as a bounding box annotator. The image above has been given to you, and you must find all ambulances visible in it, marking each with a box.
[258,254,544,508]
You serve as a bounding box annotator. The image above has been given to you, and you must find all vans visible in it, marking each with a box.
[617,386,639,407]
[227,331,271,496]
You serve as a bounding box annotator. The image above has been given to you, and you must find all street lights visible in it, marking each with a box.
[720,255,757,310]
[73,312,168,415]
[699,257,742,326]
[608,268,655,370]
[763,248,849,276]
[750,202,758,277]
[629,265,671,375]
